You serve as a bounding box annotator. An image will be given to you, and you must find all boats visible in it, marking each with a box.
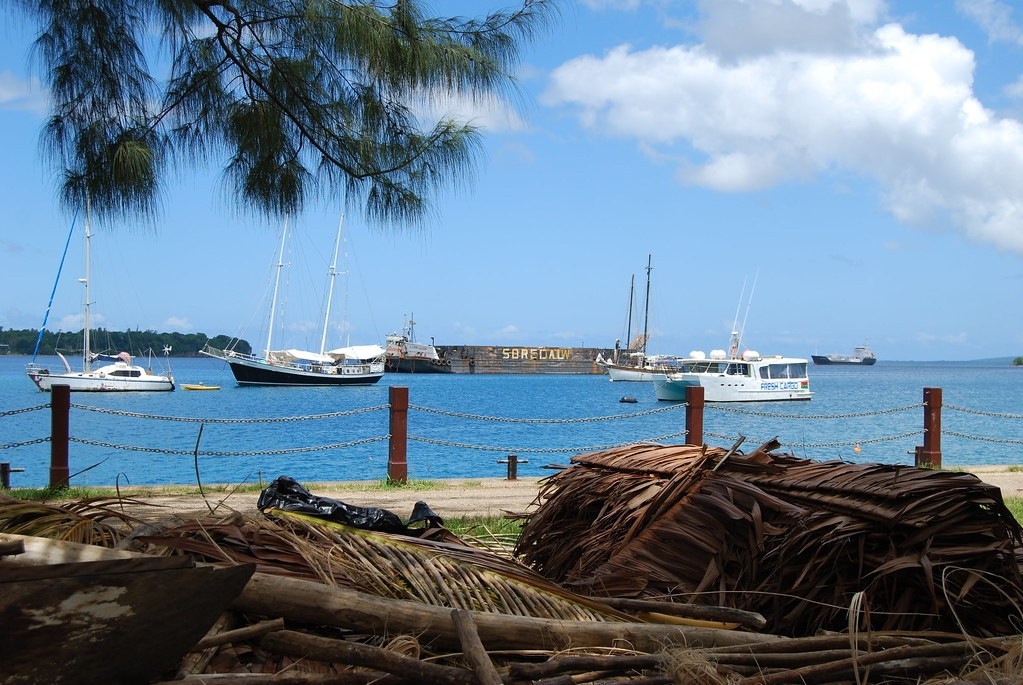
[648,256,816,405]
[812,337,877,367]
[179,381,221,392]
[383,311,454,374]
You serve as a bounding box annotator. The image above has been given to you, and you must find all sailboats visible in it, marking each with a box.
[197,208,385,386]
[597,256,681,383]
[23,180,178,395]
